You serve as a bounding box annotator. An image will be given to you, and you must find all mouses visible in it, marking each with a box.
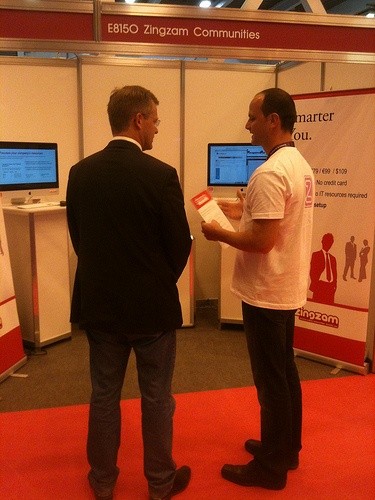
[60,201,66,206]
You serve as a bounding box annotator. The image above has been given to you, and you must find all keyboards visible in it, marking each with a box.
[17,202,59,209]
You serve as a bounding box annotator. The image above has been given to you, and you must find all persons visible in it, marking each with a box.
[201,87,316,490]
[66,86,191,500]
[342,235,357,281]
[358,240,371,283]
[309,232,338,304]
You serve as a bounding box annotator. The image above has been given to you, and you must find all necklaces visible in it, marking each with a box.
[266,141,295,160]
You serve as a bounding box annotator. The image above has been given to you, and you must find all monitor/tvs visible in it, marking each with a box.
[0,141,60,203]
[207,143,269,192]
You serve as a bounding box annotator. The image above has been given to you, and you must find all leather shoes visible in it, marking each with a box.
[244,439,300,470]
[153,465,190,500]
[88,467,113,500]
[220,463,289,491]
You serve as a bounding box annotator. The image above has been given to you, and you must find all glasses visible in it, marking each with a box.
[143,111,161,126]
